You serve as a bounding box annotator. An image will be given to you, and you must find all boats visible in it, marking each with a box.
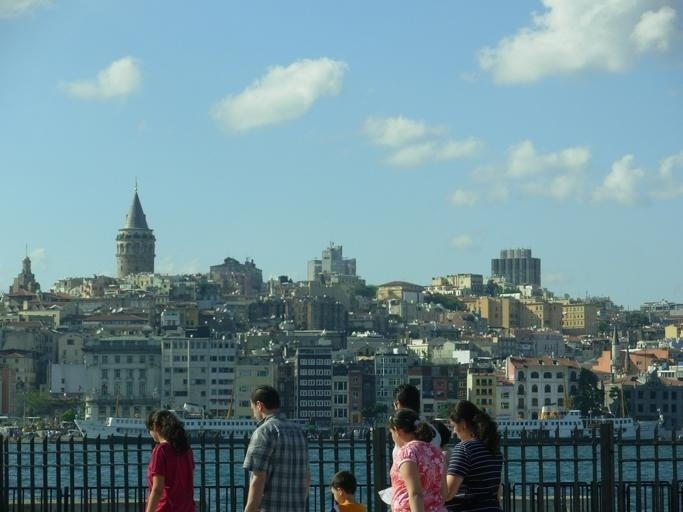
[423,410,663,445]
[73,416,318,444]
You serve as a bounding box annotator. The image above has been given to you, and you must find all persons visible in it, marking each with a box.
[143,410,195,512]
[389,407,448,512]
[328,470,368,512]
[392,383,442,451]
[429,421,451,475]
[445,399,504,512]
[242,385,311,512]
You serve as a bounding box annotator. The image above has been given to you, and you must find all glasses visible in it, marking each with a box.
[392,400,400,407]
